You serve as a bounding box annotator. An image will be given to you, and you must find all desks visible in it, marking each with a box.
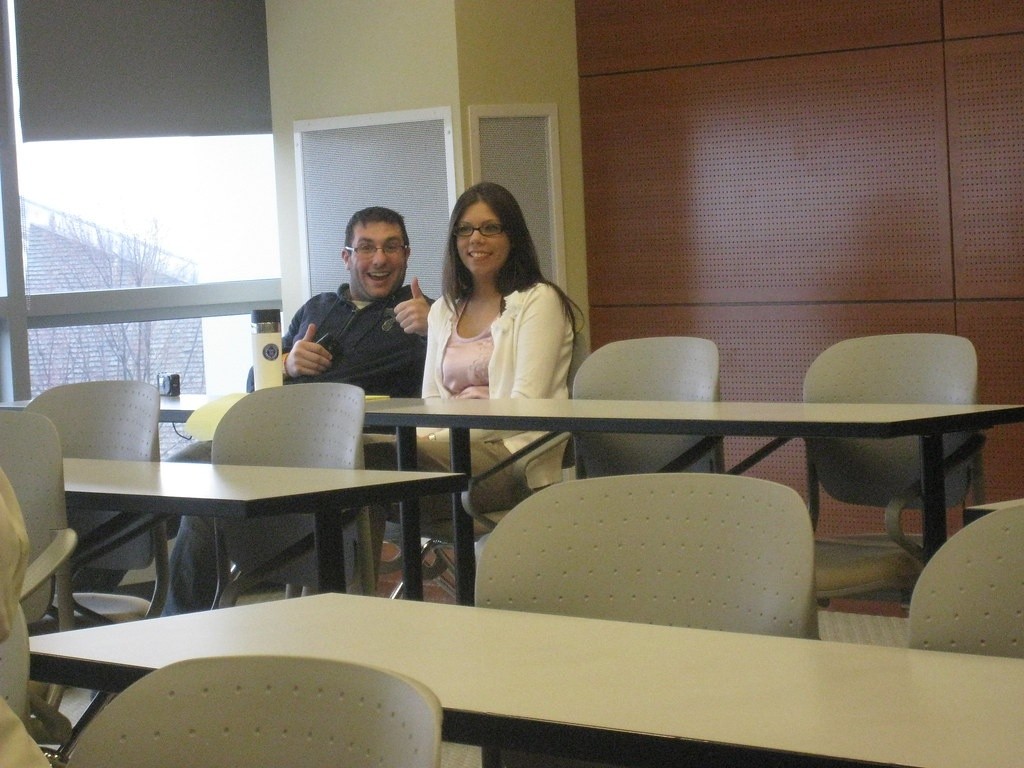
[62,457,470,615]
[26,591,1024,768]
[0,395,1024,606]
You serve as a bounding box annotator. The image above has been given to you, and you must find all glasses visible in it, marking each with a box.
[453,223,503,237]
[345,243,408,258]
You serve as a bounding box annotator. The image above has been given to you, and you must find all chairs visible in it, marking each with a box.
[0,337,1024,768]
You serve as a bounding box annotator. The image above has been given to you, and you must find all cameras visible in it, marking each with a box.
[157,371,180,396]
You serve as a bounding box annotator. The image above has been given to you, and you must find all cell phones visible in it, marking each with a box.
[315,333,343,358]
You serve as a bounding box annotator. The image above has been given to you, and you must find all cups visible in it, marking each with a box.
[250,309,284,392]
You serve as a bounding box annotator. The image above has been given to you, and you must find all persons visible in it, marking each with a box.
[159,206,436,617]
[391,183,576,526]
